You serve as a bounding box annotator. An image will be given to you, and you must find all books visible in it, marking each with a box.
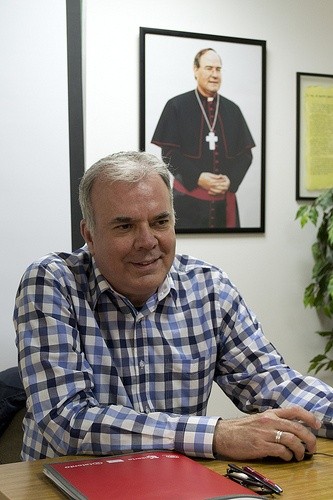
[40,449,271,500]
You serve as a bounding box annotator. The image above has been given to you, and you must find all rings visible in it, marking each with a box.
[274,430,283,443]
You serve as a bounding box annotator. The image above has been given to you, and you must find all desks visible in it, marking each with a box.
[0,428,333,500]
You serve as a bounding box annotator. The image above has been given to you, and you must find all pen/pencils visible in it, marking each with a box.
[243,465,283,493]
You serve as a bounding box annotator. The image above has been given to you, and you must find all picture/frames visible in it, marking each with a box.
[294,72,333,199]
[141,27,267,236]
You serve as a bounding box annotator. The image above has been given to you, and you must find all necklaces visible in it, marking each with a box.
[195,87,220,150]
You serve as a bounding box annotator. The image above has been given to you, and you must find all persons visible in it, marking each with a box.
[150,47,257,228]
[13,152,333,463]
